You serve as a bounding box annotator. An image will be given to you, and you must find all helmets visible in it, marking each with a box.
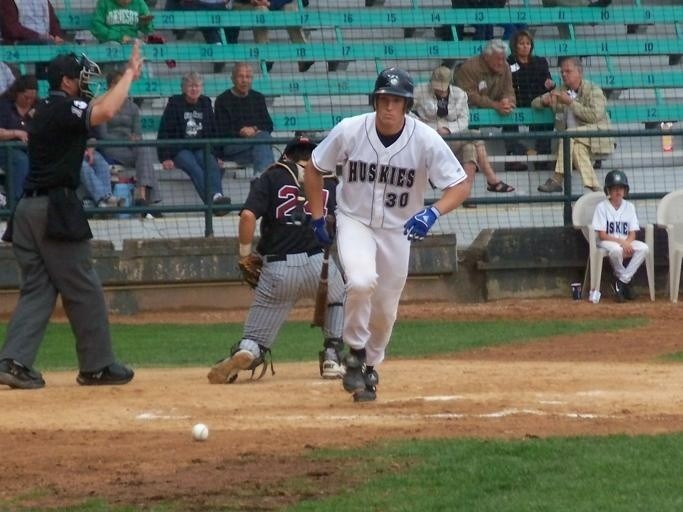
[374,67,414,99]
[47,52,102,101]
[603,170,628,196]
[279,135,318,164]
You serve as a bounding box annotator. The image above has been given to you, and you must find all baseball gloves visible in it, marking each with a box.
[238,254,262,289]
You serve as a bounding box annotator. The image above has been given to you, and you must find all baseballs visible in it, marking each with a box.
[194,424,208,440]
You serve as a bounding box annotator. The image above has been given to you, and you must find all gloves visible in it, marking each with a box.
[311,216,334,246]
[402,205,440,244]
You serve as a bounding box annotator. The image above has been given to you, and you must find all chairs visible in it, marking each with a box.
[572,191,655,304]
[656,189,683,303]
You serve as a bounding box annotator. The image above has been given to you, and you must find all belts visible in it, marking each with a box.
[267,248,323,263]
[22,190,49,198]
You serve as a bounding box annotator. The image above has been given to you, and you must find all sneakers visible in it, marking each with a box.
[0,359,44,390]
[611,279,625,303]
[77,364,134,386]
[537,178,562,192]
[342,359,365,394]
[97,196,230,220]
[624,283,636,300]
[353,369,378,402]
[321,360,347,380]
[207,348,258,385]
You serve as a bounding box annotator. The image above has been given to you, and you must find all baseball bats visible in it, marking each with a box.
[313,215,336,327]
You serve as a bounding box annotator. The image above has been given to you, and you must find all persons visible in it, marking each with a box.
[592,171,648,302]
[0,36,143,390]
[90,0,165,71]
[156,72,232,217]
[80,72,163,219]
[168,1,315,74]
[408,31,613,209]
[1,62,42,208]
[215,63,275,173]
[207,135,346,385]
[305,67,471,401]
[2,0,64,46]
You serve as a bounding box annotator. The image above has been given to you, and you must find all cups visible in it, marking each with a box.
[572,284,582,301]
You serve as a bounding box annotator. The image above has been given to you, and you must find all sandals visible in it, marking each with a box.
[486,181,514,192]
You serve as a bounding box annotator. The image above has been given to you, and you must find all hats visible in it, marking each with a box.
[431,66,451,91]
